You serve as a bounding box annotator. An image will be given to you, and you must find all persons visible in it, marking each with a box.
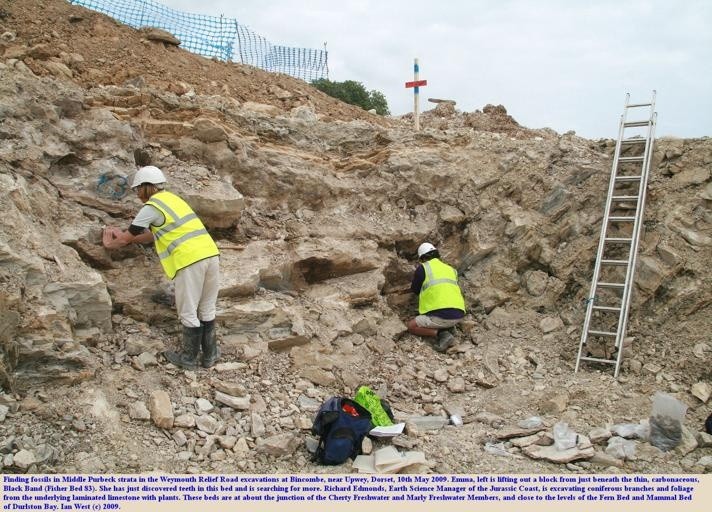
[102,164,223,372]
[405,242,468,353]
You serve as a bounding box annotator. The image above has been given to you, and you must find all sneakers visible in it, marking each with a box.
[434,330,454,352]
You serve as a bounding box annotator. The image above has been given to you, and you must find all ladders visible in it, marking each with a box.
[575,90,657,378]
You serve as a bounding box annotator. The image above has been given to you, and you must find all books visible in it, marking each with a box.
[369,421,407,438]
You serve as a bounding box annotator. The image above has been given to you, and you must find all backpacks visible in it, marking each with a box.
[312,395,372,466]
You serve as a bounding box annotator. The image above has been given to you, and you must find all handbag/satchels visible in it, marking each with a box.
[354,386,394,434]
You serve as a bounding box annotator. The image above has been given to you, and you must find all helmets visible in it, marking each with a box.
[417,242,438,258]
[130,166,167,189]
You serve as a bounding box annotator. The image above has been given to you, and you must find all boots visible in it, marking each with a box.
[200,320,222,368]
[164,323,203,369]
